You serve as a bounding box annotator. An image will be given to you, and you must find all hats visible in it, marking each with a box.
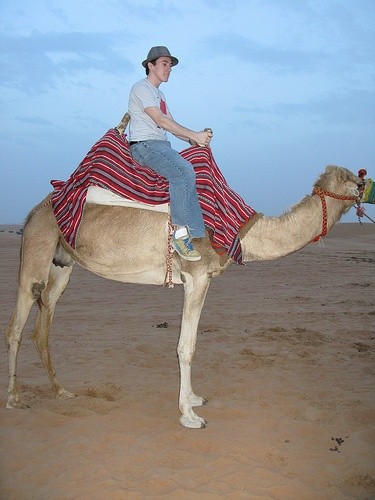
[142,47,179,66]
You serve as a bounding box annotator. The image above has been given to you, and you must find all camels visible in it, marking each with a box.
[1,111,375,431]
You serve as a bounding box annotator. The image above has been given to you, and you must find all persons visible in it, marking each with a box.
[127,47,212,262]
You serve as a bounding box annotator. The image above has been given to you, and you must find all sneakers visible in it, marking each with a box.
[170,232,201,261]
